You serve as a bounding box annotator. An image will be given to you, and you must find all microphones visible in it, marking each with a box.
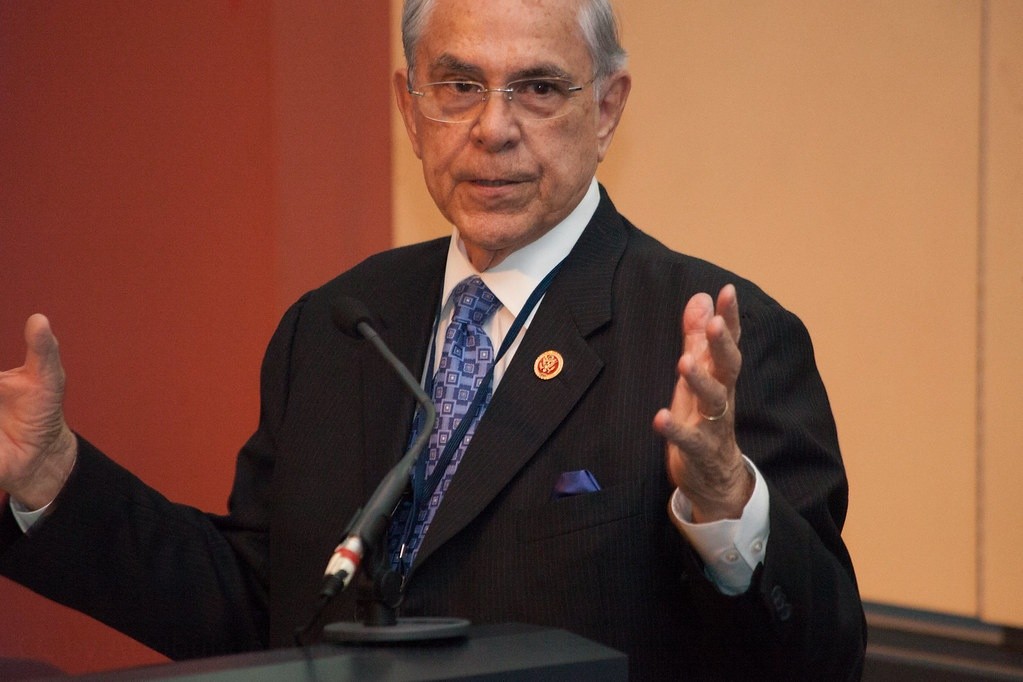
[317,298,436,605]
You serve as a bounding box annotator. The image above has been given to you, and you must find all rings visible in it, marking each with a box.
[700,402,729,421]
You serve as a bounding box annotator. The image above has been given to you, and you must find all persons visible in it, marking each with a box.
[1,0,867,682]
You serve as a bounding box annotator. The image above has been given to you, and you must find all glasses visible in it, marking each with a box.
[404,65,605,124]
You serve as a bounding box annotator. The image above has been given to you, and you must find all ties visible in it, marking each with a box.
[388,274,500,606]
